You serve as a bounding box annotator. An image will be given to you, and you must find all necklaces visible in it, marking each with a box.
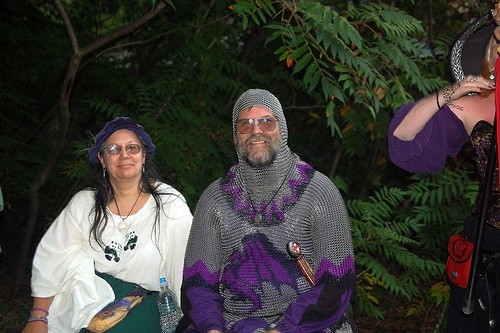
[112,182,143,234]
[247,176,287,225]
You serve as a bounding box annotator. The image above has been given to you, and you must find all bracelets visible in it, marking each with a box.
[443,86,451,104]
[28,316,48,323]
[28,307,49,315]
[436,89,441,109]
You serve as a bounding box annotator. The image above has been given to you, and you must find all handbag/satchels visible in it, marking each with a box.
[86,294,144,333]
[445,232,475,292]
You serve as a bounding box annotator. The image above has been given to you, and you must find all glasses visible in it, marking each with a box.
[235,116,277,134]
[103,143,143,155]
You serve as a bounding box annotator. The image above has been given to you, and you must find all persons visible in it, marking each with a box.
[388,0,500,333]
[181,89,356,333]
[22,116,194,333]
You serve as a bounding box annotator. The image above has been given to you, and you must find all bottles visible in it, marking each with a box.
[158,277,182,333]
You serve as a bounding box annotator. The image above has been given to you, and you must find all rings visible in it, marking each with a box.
[466,74,480,81]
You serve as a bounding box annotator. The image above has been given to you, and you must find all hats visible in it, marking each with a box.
[446,11,497,85]
[88,117,155,164]
